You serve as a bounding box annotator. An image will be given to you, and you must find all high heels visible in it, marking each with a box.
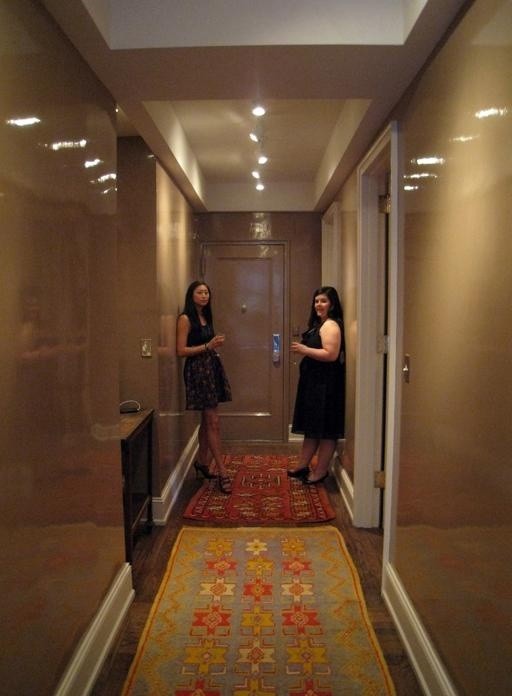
[218,474,233,493]
[287,467,330,485]
[194,460,218,479]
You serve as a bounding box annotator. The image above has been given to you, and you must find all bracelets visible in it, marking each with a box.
[204,343,210,353]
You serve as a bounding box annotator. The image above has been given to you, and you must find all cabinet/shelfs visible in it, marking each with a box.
[119,407,156,574]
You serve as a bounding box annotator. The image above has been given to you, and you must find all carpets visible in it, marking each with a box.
[182,454,337,525]
[118,525,397,696]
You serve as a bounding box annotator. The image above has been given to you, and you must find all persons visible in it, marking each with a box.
[158,283,177,414]
[16,267,87,486]
[177,282,235,495]
[285,285,345,486]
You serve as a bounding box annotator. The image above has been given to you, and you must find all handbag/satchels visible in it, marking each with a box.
[120,400,144,412]
